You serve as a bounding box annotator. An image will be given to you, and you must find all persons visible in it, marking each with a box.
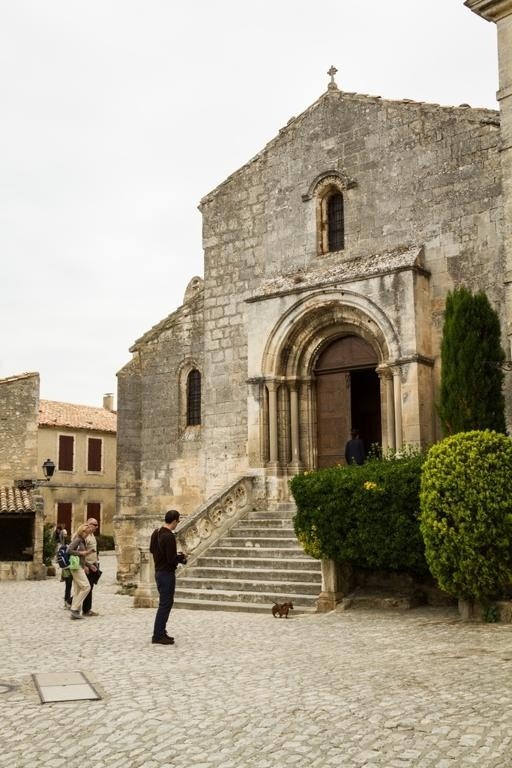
[345,430,365,465]
[149,508,187,646]
[64,521,92,620]
[54,523,73,610]
[79,517,99,618]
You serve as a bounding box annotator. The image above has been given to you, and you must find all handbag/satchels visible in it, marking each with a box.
[66,554,81,571]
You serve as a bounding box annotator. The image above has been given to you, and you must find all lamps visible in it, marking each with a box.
[37,458,56,482]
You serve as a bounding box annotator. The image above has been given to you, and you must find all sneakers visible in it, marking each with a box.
[82,611,99,616]
[70,612,84,620]
[152,638,175,644]
[164,633,174,640]
[63,604,70,610]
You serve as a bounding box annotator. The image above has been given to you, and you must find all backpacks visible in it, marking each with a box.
[57,544,70,567]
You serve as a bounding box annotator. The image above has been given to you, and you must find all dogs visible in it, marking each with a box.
[271,600,293,618]
